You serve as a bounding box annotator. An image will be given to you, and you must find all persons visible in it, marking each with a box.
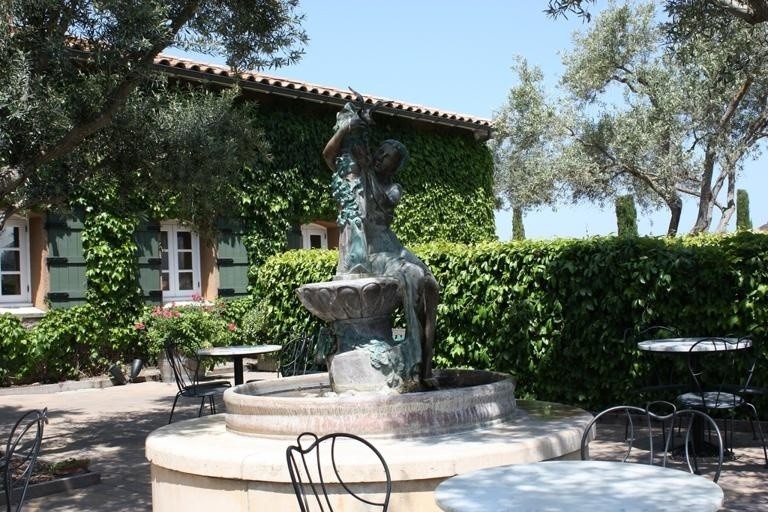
[323,109,441,389]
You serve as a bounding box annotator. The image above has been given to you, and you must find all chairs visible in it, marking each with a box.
[0,407,48,512]
[624,325,768,475]
[287,433,391,512]
[580,400,724,484]
[165,330,309,424]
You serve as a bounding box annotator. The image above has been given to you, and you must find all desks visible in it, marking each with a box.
[434,460,725,512]
